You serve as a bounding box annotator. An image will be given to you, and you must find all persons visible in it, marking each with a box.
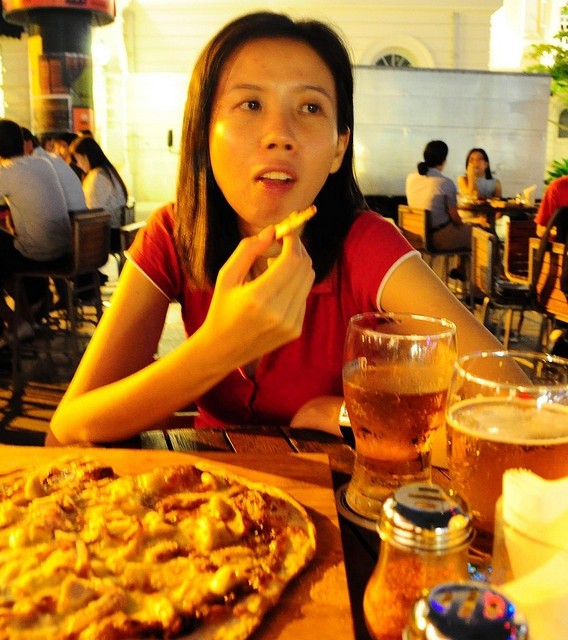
[68,136,127,285]
[404,140,482,303]
[34,131,84,182]
[457,148,501,198]
[19,126,86,227]
[532,176,567,239]
[48,10,537,445]
[0,119,73,343]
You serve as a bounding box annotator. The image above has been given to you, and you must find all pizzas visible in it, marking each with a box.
[272,205,315,246]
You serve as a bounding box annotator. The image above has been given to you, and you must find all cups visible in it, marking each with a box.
[342,315,455,528]
[490,494,568,638]
[447,352,567,568]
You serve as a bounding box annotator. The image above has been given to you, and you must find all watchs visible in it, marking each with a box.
[339,397,356,450]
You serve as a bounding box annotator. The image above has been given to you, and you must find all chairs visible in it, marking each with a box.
[467,226,552,351]
[101,204,134,277]
[397,204,467,302]
[528,237,568,378]
[495,214,535,341]
[10,208,112,378]
